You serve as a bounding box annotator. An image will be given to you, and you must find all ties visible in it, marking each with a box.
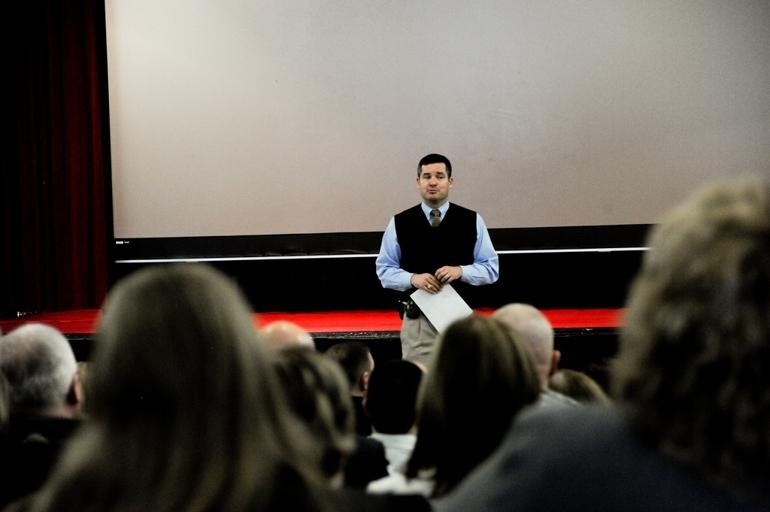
[430,209,441,228]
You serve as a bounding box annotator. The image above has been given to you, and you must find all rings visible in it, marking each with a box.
[428,285,433,289]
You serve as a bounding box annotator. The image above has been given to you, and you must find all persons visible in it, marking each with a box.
[408,180,770,512]
[1,261,434,512]
[375,151,497,377]
